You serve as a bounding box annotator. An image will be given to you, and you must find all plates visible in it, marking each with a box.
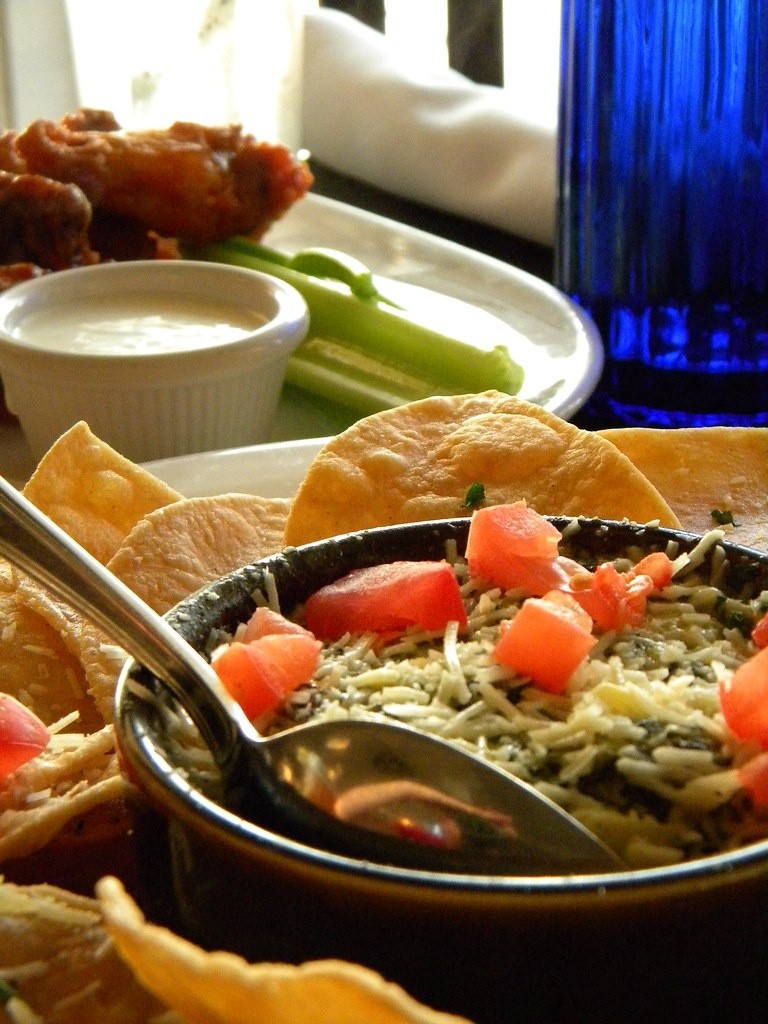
[130,434,333,500]
[1,189,603,489]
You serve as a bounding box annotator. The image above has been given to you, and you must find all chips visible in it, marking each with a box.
[0,389,768,1024]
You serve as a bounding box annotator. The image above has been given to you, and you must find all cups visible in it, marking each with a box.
[557,0,768,426]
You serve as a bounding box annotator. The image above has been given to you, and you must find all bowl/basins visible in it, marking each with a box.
[1,260,310,470]
[109,514,768,938]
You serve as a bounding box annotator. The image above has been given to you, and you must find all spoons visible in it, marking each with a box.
[0,476,626,877]
